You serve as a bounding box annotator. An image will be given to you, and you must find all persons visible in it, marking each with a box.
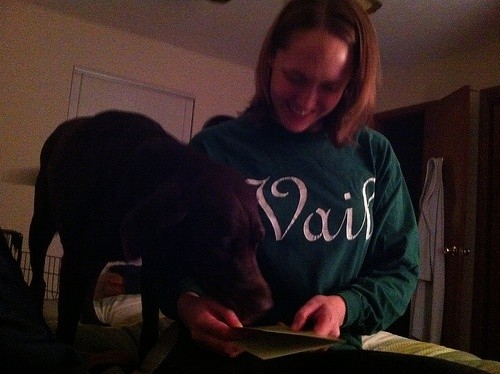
[157,0,421,372]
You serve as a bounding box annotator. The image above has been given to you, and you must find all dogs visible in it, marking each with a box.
[30,110,277,366]
